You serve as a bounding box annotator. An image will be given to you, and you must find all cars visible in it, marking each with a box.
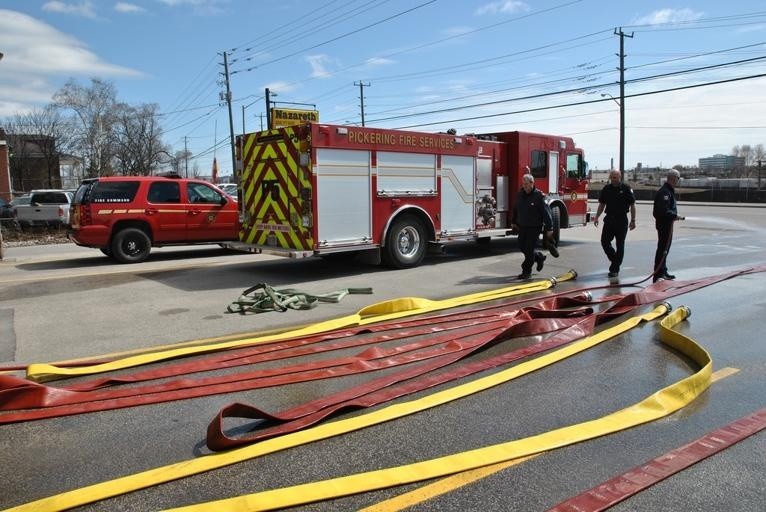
[0,189,75,230]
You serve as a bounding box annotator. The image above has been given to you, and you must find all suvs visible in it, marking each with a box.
[68,177,239,263]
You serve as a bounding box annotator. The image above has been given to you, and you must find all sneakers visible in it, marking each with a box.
[519,274,531,279]
[608,253,620,277]
[653,272,676,284]
[537,256,546,271]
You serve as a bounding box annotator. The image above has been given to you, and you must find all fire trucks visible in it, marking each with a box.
[236,121,590,270]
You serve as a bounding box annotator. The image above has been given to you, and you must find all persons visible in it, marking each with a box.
[593,169,636,277]
[510,174,553,278]
[649,169,685,283]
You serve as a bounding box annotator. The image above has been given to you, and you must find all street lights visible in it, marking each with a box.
[601,91,625,182]
